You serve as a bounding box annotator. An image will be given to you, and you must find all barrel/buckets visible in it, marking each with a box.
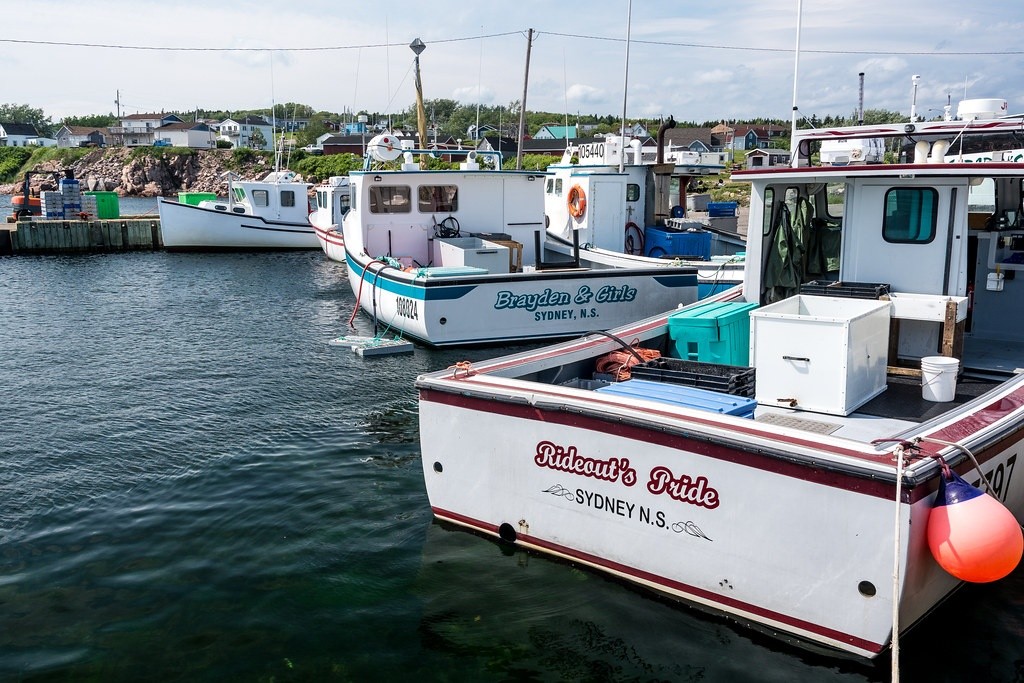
[919,356,960,402]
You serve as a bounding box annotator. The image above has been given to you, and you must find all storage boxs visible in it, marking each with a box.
[645,226,712,262]
[178,192,216,206]
[433,237,509,274]
[707,202,738,216]
[40,178,119,220]
[555,281,892,421]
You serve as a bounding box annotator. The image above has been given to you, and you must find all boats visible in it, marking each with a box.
[156,90,1024,675]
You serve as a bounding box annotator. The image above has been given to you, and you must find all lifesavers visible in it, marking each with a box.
[567,183,586,217]
